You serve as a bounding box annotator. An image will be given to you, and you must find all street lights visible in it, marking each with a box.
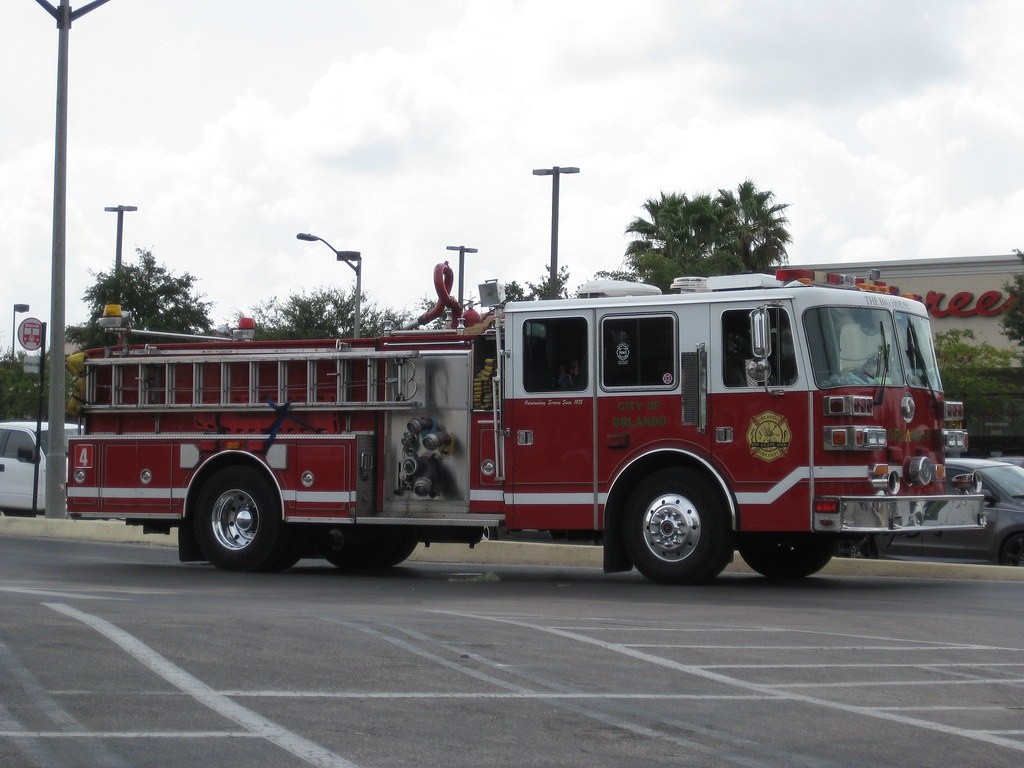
[296,233,363,340]
[10,303,31,359]
[103,204,138,306]
[445,244,479,316]
[532,166,582,298]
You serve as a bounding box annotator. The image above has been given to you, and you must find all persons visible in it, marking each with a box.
[541,362,570,390]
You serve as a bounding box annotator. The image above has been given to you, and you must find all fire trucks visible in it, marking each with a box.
[65,268,988,585]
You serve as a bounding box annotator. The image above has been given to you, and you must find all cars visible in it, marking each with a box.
[0,422,85,513]
[830,459,1024,567]
